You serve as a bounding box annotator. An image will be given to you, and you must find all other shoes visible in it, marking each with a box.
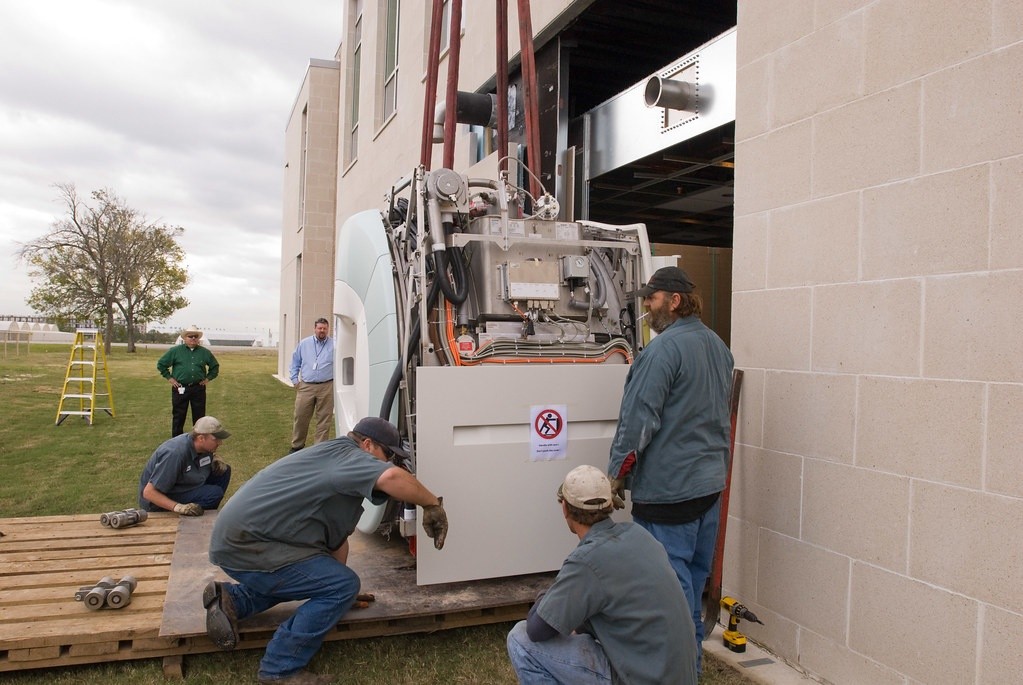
[290,443,306,455]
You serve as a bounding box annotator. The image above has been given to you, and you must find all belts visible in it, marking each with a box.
[179,382,200,387]
[303,378,333,384]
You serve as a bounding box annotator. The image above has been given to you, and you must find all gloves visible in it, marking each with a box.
[603,473,628,510]
[347,592,376,610]
[422,496,449,550]
[211,454,228,477]
[173,503,204,516]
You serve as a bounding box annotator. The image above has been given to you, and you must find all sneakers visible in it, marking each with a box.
[257,672,328,685]
[202,580,239,649]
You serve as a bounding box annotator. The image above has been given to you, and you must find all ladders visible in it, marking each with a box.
[55,328,116,426]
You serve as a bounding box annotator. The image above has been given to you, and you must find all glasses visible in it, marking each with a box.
[361,437,396,462]
[185,334,198,338]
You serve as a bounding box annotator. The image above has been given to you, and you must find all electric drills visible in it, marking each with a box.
[718,596,765,653]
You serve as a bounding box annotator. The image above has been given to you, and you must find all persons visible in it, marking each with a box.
[138,416,231,516]
[203,416,448,685]
[157,327,220,438]
[608,266,734,673]
[290,318,334,454]
[506,464,698,685]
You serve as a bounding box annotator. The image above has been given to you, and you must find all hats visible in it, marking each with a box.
[353,417,410,459]
[193,415,232,439]
[557,465,611,509]
[636,266,693,298]
[181,325,202,340]
